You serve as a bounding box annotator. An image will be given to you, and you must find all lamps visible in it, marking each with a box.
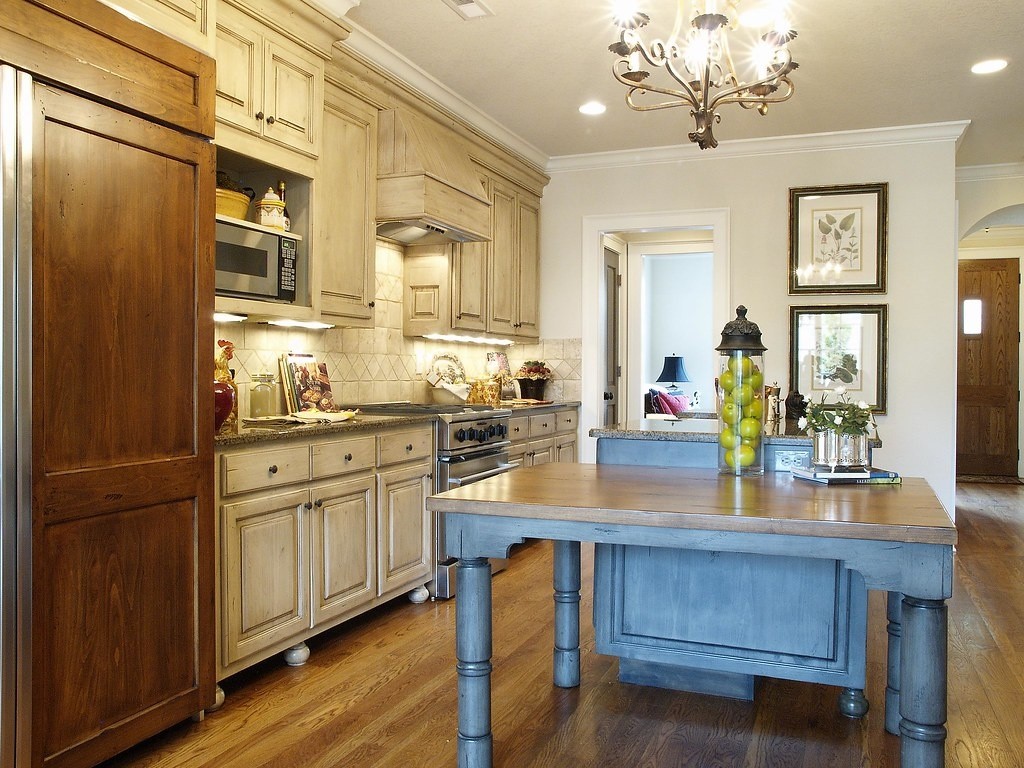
[608,0,800,149]
[655,352,692,387]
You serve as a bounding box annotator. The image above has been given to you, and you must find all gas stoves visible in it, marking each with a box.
[335,399,513,451]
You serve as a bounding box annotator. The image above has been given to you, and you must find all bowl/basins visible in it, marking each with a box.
[429,386,466,405]
[215,188,249,220]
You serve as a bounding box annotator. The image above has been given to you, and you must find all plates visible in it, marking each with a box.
[430,351,467,386]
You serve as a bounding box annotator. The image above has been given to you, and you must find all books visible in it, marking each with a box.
[793,460,904,487]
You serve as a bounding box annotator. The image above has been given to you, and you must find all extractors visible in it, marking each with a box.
[374,216,491,247]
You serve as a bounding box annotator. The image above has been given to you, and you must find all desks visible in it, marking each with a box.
[427,464,959,767]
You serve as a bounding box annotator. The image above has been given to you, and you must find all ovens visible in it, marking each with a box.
[426,440,519,601]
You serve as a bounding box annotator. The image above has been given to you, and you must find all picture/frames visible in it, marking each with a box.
[787,303,887,415]
[787,181,890,297]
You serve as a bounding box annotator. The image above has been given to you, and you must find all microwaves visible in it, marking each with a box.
[213,221,297,305]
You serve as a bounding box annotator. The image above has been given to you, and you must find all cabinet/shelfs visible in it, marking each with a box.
[0,0,216,768]
[215,12,327,159]
[555,406,578,464]
[320,77,379,329]
[403,160,489,336]
[101,0,217,54]
[488,178,541,345]
[508,412,553,475]
[213,429,376,683]
[378,422,436,607]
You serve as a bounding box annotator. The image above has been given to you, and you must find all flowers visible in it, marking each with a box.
[514,360,553,378]
[796,386,876,440]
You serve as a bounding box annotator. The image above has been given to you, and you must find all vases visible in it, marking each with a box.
[518,379,548,401]
[813,429,869,467]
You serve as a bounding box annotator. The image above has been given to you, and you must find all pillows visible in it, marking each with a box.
[644,388,700,415]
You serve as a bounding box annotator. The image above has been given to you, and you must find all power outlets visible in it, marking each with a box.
[774,450,808,471]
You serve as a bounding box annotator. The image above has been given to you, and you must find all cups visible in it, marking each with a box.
[467,376,501,409]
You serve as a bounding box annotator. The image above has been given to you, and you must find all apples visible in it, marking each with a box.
[719,355,763,467]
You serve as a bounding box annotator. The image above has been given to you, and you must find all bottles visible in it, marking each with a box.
[715,309,769,477]
[249,373,276,417]
[275,180,292,233]
[220,369,238,435]
